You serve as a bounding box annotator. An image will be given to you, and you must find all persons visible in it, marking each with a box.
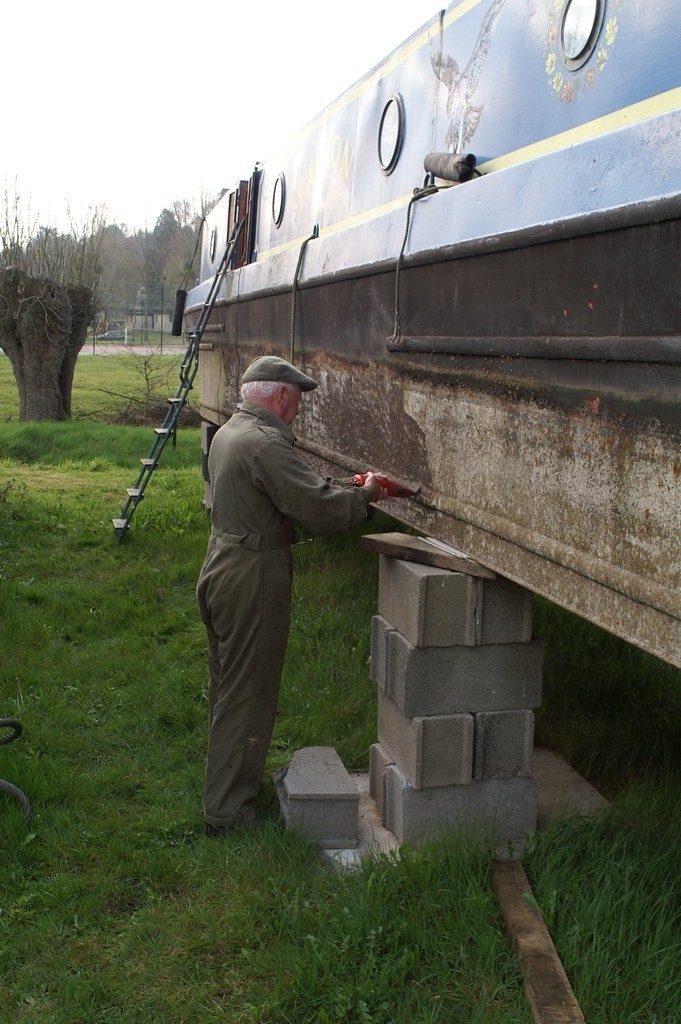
[195,356,387,839]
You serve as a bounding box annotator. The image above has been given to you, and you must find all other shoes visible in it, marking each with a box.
[205,822,243,838]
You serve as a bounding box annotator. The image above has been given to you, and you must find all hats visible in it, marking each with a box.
[240,355,318,392]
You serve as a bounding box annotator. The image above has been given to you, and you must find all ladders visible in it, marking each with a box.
[108,213,256,548]
